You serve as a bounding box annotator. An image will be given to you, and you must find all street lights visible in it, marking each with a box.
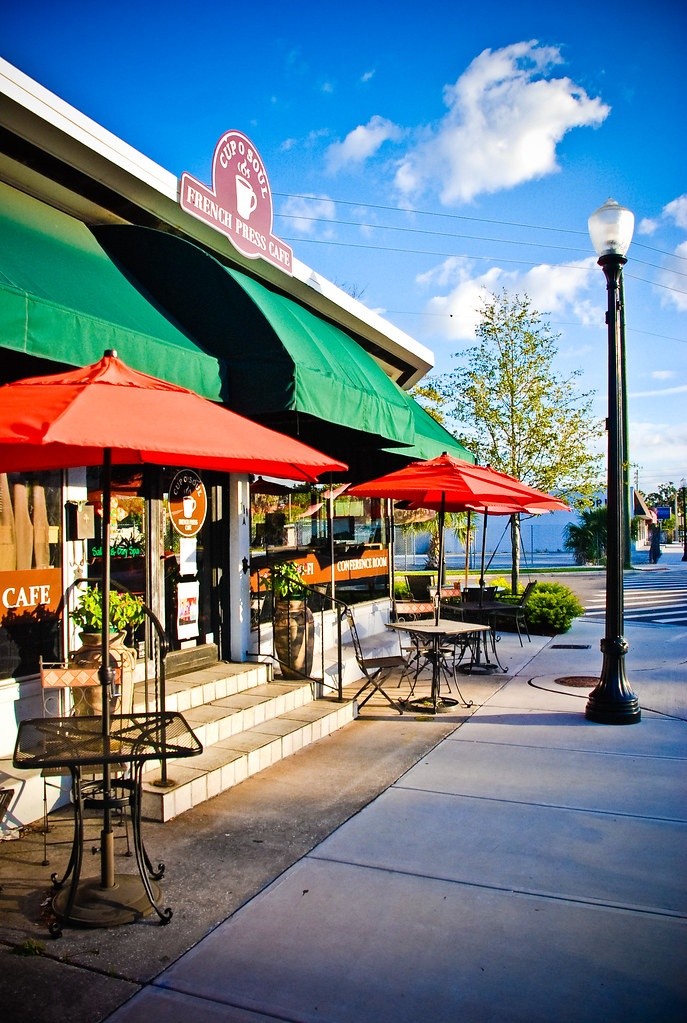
[681,478,687,561]
[584,199,644,725]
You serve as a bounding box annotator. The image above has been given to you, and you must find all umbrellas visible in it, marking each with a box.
[340,452,571,700]
[0,350,348,886]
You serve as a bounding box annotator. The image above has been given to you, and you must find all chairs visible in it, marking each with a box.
[38,657,134,866]
[345,575,537,714]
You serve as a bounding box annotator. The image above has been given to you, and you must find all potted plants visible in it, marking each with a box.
[68,586,144,733]
[263,563,314,680]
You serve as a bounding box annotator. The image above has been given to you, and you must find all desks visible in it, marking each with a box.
[13,712,203,938]
[384,618,492,707]
[440,600,522,673]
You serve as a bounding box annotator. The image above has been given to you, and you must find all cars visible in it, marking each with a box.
[660,529,672,544]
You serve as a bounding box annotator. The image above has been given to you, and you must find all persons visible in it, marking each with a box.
[648,523,660,564]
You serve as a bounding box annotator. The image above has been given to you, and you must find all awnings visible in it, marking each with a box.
[0,184,222,403]
[377,389,475,468]
[93,220,475,470]
[635,491,652,520]
